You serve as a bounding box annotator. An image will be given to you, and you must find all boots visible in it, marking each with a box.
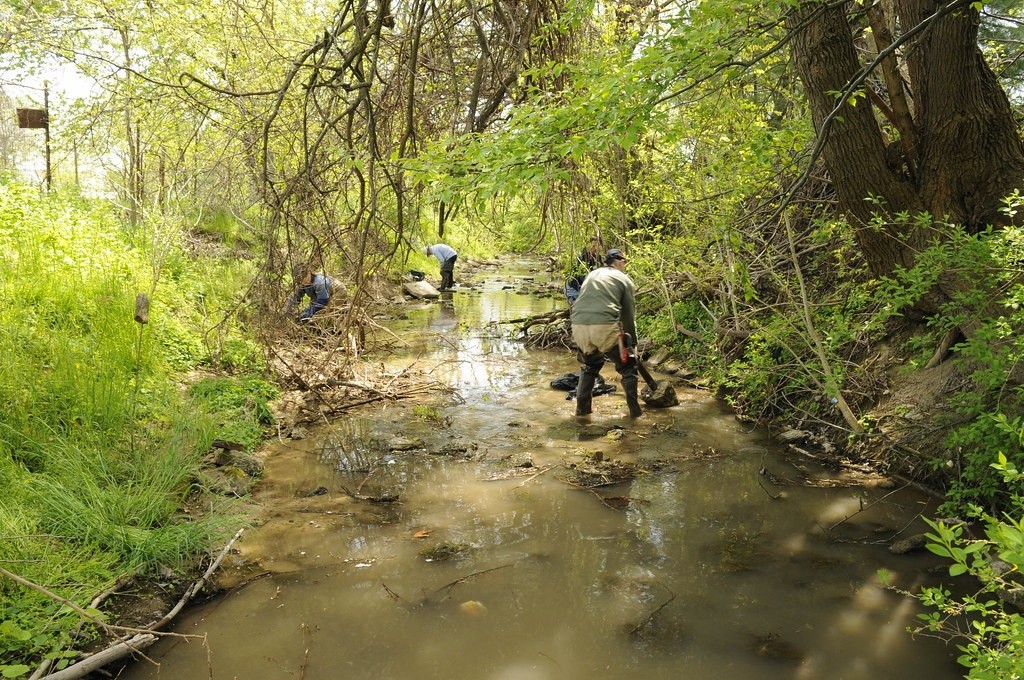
[576,373,595,415]
[621,376,642,419]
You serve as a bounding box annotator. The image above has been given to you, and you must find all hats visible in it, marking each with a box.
[605,249,621,260]
[422,246,429,258]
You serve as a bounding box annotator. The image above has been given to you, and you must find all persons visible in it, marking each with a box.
[285,263,347,320]
[577,235,604,271]
[570,249,644,418]
[421,244,457,291]
